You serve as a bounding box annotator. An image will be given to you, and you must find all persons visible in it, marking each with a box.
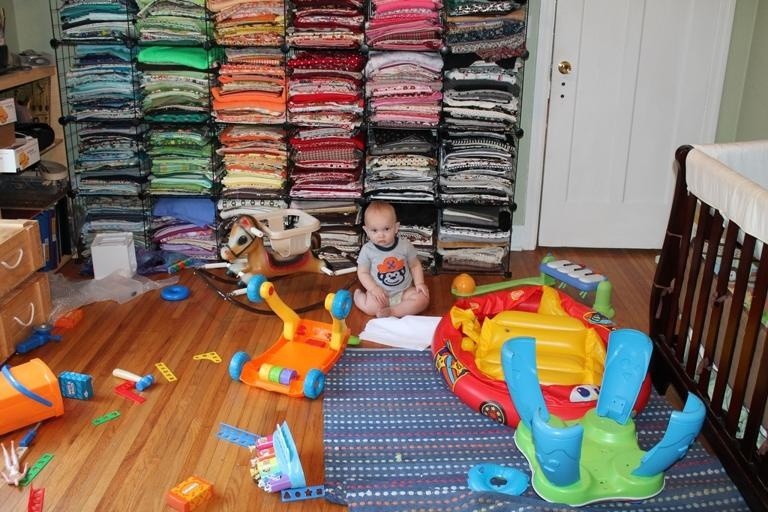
[353,199,430,319]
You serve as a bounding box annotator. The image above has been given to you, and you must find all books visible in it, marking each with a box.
[357,311,443,352]
[696,241,768,331]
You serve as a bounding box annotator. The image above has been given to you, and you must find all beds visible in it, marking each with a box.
[644,137,767,512]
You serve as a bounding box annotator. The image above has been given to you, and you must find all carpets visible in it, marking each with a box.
[321,347,752,512]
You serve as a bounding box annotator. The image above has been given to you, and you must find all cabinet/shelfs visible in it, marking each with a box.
[2,61,76,366]
